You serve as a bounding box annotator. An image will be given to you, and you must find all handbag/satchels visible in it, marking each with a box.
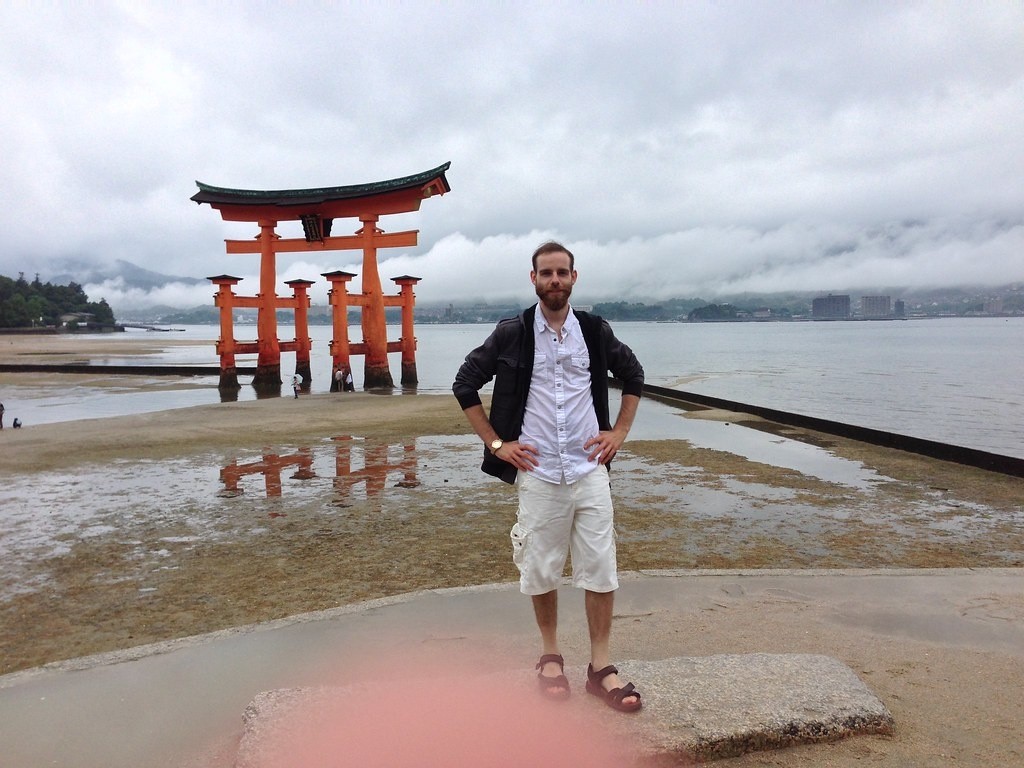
[296,384,301,391]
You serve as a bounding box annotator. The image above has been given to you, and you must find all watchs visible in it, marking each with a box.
[490,439,503,456]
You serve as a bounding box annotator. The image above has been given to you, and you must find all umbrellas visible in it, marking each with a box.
[291,374,303,384]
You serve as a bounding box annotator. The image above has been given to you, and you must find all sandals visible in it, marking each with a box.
[585,663,642,712]
[535,654,571,702]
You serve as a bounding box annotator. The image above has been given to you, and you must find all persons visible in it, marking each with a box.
[0,402,5,428]
[335,369,345,392]
[292,376,299,399]
[13,418,22,428]
[345,371,353,392]
[452,242,644,711]
